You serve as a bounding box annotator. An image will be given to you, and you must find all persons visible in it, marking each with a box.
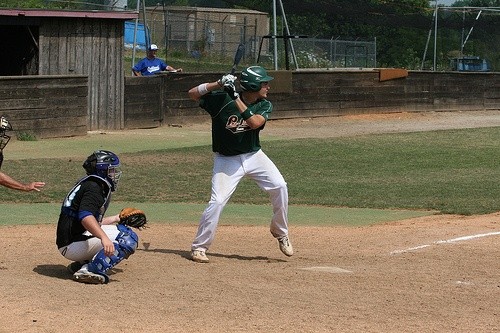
[132,44,183,76]
[188,66,294,263]
[57,149,148,284]
[0,118,46,193]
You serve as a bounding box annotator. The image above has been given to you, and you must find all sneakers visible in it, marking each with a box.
[270,229,293,257]
[189,248,209,263]
[73,265,106,284]
[68,261,82,274]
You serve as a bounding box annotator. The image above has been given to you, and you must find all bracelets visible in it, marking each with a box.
[239,108,254,121]
[198,83,210,95]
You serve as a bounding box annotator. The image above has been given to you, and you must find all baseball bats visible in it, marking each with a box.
[224,43,246,93]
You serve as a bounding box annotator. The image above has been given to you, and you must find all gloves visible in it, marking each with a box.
[223,80,239,100]
[217,74,238,88]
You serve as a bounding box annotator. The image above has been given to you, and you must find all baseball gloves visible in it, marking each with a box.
[119,208,147,227]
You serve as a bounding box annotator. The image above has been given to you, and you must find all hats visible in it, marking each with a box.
[148,44,159,50]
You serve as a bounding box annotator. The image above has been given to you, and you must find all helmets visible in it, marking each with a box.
[239,65,275,92]
[82,150,121,191]
[0,116,13,150]
